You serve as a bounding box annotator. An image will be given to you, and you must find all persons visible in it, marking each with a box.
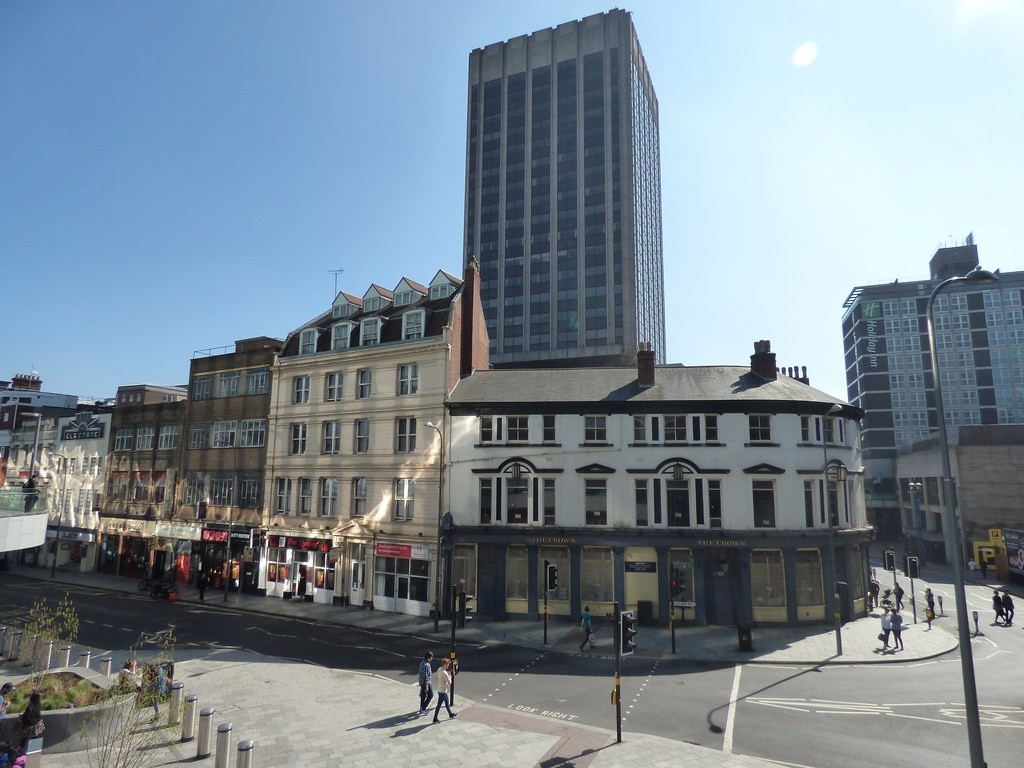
[432,657,459,723]
[118,658,166,723]
[579,606,597,652]
[419,651,434,716]
[23,474,37,513]
[968,558,987,579]
[868,579,904,613]
[197,573,209,601]
[992,589,1015,627]
[0,682,40,768]
[168,562,178,588]
[881,608,904,651]
[924,588,935,619]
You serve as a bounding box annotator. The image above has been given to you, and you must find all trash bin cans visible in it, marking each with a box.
[142,657,175,705]
[738,625,752,650]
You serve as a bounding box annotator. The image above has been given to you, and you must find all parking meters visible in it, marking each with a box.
[926,608,933,630]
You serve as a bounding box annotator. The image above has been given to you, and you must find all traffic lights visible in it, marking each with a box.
[623,615,638,654]
[464,595,475,624]
[672,568,687,596]
[549,567,559,591]
[888,553,894,571]
[910,559,920,579]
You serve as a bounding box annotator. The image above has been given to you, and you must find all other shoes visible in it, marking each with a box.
[451,713,458,719]
[579,646,583,652]
[420,708,429,715]
[433,719,442,723]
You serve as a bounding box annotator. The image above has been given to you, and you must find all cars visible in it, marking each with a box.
[25,550,36,566]
[71,549,81,562]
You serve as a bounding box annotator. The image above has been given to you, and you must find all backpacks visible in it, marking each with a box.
[897,587,904,594]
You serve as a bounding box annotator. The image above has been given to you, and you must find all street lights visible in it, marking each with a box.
[424,419,443,634]
[47,451,68,579]
[924,265,1001,768]
[822,403,850,655]
[213,438,236,603]
[21,411,41,490]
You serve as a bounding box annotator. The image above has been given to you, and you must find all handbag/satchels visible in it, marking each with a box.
[588,633,597,646]
[878,633,887,643]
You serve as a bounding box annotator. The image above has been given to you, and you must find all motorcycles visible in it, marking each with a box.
[150,583,178,601]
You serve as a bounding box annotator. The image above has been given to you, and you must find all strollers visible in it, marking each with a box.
[879,591,896,608]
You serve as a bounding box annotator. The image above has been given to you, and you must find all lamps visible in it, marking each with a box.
[836,463,847,481]
[512,461,521,479]
[674,462,684,481]
